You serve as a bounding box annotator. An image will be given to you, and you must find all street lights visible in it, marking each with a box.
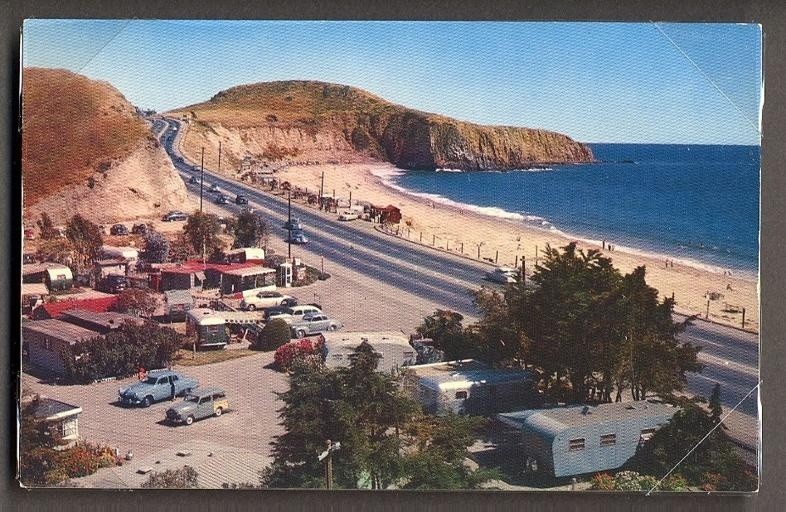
[280,189,299,257]
[315,437,344,490]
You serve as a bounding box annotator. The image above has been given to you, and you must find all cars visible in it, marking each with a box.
[160,114,309,247]
[338,211,360,222]
[484,265,521,285]
[23,222,148,239]
[93,274,129,295]
[115,284,342,426]
